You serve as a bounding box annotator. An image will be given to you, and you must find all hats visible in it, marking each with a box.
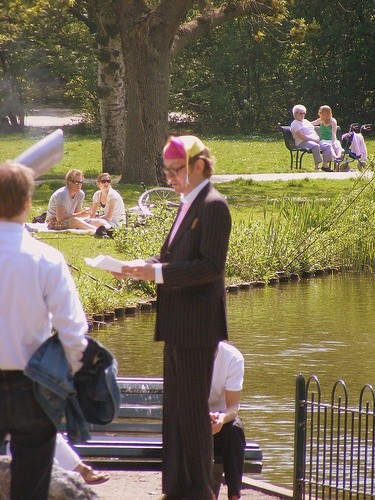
[164,134,205,185]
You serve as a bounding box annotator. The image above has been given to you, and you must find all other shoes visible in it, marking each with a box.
[107,229,115,238]
[80,468,110,484]
[95,226,105,238]
[321,167,333,172]
[315,166,322,171]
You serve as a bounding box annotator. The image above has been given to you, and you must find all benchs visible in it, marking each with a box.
[278,122,345,170]
[1,377,262,500]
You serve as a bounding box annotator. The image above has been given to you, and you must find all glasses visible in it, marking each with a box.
[162,157,199,176]
[69,179,83,185]
[96,180,111,183]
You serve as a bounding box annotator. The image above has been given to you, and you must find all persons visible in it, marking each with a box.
[207,341,247,500]
[0,160,89,500]
[290,104,341,172]
[84,173,127,230]
[45,170,112,230]
[104,136,232,500]
[0,431,111,486]
